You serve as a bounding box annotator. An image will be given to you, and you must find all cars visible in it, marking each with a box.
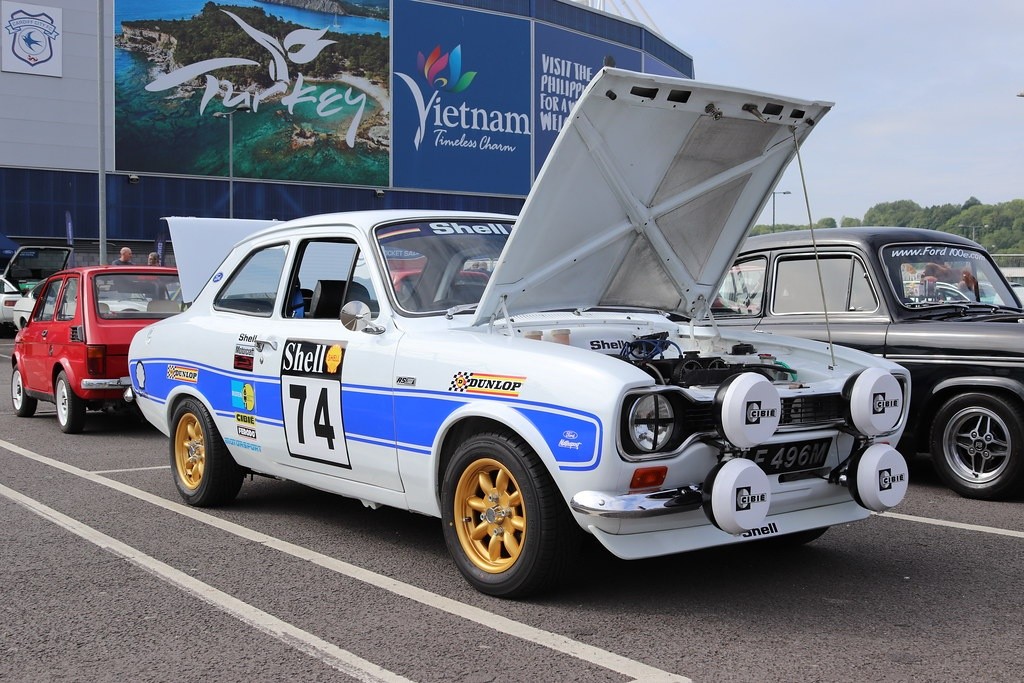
[707,226,1024,500]
[903,281,971,305]
[128,65,913,600]
[10,264,190,435]
[14,275,149,330]
[0,245,75,336]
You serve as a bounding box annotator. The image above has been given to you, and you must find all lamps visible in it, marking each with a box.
[376,189,385,198]
[129,174,140,184]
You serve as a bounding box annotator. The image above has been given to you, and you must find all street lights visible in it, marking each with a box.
[212,109,238,219]
[771,191,792,232]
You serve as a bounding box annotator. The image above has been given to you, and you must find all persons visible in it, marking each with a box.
[465,262,492,277]
[148,252,160,266]
[112,247,133,265]
[903,263,969,300]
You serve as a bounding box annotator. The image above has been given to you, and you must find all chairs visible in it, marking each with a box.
[226,248,305,321]
[310,279,370,319]
[146,299,181,314]
[96,301,111,315]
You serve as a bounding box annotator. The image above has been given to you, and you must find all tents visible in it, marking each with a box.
[0,234,21,258]
